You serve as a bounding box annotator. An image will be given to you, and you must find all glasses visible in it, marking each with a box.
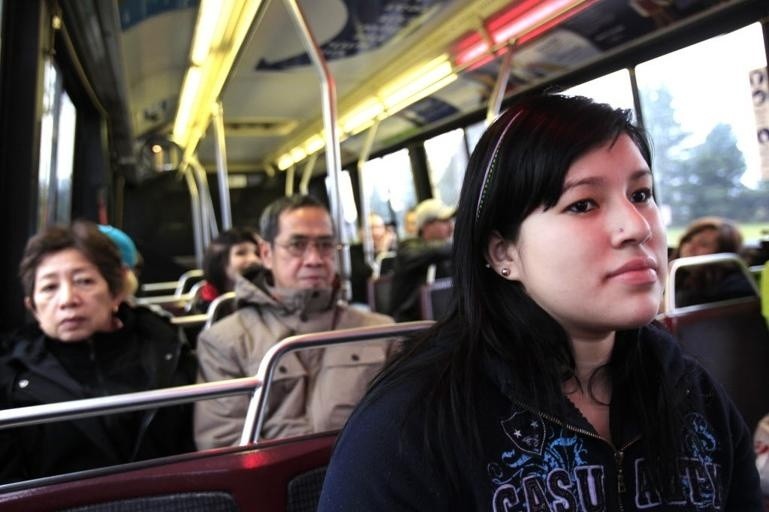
[277,238,346,261]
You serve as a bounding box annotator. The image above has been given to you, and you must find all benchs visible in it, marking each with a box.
[2,249,769,510]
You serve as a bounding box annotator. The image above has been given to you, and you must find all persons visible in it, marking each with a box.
[187,227,267,338]
[344,202,417,315]
[315,88,766,512]
[389,197,459,323]
[192,191,409,454]
[754,414,769,500]
[667,219,757,307]
[94,224,190,347]
[1,219,198,485]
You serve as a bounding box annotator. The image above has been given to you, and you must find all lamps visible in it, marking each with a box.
[162,0,598,172]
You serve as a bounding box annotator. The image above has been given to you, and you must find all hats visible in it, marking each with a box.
[411,198,458,236]
[95,224,142,269]
